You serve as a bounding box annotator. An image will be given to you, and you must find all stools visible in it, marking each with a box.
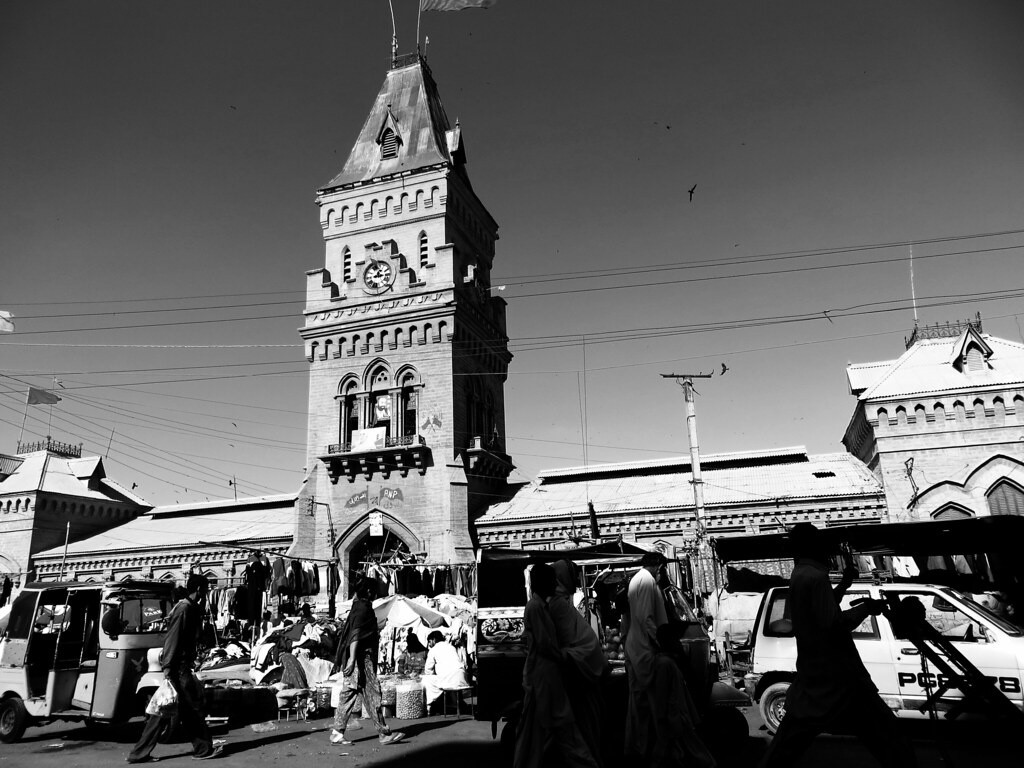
[440,686,475,720]
[277,693,307,723]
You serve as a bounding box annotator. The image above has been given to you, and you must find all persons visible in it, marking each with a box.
[622,552,716,768]
[763,524,914,768]
[421,630,470,715]
[513,559,613,768]
[128,574,223,763]
[330,580,405,745]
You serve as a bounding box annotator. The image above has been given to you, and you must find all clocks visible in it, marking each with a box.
[364,260,392,290]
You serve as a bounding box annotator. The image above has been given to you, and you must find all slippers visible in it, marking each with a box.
[330,729,352,747]
[191,749,223,759]
[142,757,160,763]
[379,730,406,745]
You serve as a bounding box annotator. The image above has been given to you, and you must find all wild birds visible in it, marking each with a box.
[720,362,730,376]
[228,480,233,486]
[226,443,234,449]
[232,422,237,428]
[687,184,698,203]
[174,488,211,504]
[131,482,138,490]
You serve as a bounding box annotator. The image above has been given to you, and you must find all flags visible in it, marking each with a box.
[28,388,62,405]
[420,0,498,11]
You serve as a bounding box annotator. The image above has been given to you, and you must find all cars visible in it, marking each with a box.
[463,530,755,761]
[744,578,1024,739]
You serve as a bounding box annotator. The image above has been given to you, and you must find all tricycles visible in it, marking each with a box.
[0,574,180,742]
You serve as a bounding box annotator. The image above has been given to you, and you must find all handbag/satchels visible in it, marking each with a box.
[143,676,189,718]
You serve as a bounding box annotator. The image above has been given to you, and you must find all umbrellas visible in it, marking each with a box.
[340,596,452,666]
